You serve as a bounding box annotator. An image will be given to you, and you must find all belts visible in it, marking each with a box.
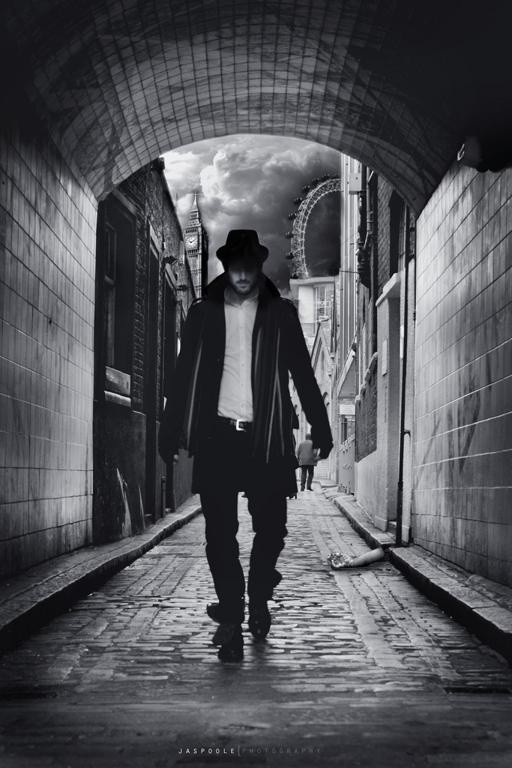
[217,416,252,431]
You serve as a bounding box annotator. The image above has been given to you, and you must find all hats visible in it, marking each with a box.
[216,228,270,265]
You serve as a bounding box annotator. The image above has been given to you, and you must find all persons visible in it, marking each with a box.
[162,229,333,666]
[296,432,318,492]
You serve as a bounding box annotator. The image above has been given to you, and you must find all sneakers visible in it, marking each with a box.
[218,625,244,662]
[250,594,272,643]
